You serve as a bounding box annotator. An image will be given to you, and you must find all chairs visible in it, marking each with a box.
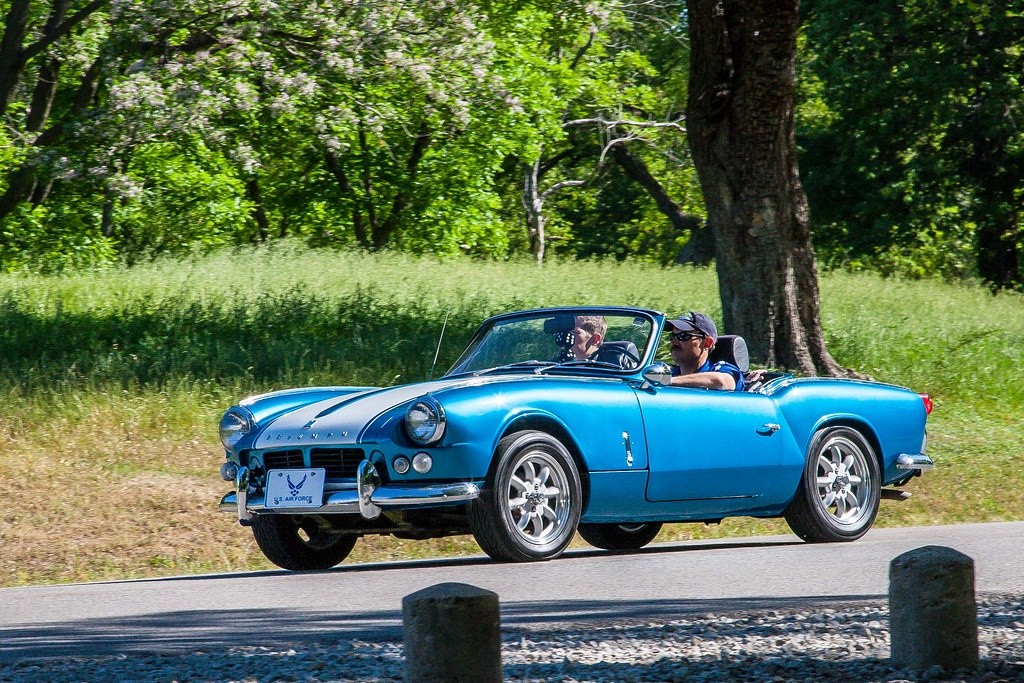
[594,341,640,370]
[708,335,749,391]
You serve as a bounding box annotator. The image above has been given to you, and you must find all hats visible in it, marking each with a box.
[663,311,718,344]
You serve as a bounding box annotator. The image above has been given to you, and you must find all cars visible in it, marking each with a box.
[218,306,934,570]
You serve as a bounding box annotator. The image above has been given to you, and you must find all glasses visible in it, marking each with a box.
[668,331,705,342]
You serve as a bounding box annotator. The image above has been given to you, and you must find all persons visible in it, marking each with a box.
[663,311,745,390]
[570,316,620,370]
